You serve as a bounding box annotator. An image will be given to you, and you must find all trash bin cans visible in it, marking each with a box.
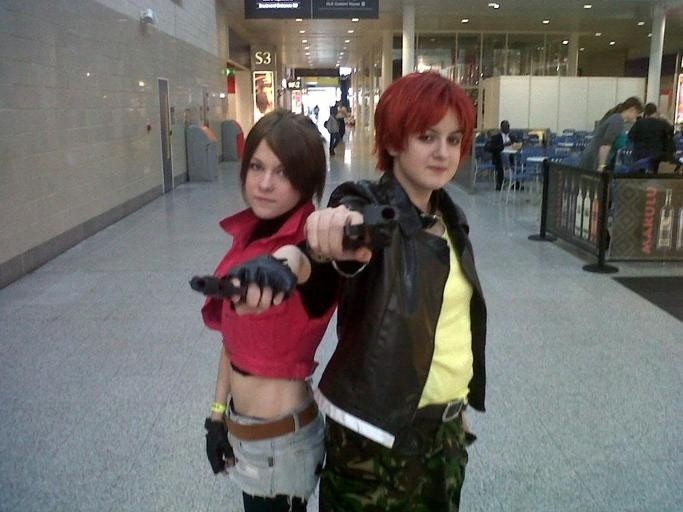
[187,124,219,181]
[221,120,244,162]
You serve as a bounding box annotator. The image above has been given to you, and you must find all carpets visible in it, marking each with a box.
[610,274,683,321]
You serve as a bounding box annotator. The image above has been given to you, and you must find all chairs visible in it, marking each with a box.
[471,129,595,205]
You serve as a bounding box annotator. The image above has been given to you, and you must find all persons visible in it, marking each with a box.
[326,106,340,155]
[608,102,683,181]
[334,100,350,140]
[577,96,643,175]
[299,68,491,511]
[196,106,344,512]
[313,104,319,122]
[482,127,497,153]
[491,119,524,191]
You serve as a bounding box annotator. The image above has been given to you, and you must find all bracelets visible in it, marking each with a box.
[207,400,229,415]
[596,160,608,169]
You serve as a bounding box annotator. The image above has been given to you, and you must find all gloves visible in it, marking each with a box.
[222,252,297,299]
[203,417,235,476]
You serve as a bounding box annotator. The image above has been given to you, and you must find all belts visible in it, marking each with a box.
[218,398,317,443]
[412,397,470,423]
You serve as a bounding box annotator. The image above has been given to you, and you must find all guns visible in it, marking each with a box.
[342,206,437,250]
[190,273,292,310]
